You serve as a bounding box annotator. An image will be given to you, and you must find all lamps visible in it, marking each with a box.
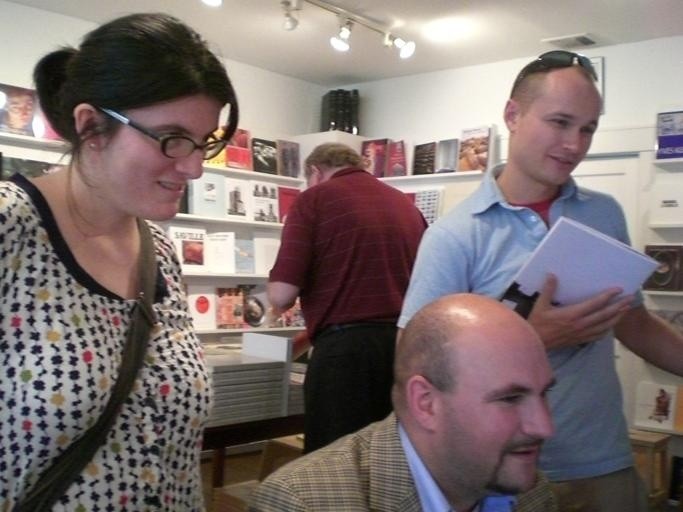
[276,1,418,60]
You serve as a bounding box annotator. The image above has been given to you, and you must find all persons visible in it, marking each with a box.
[265,141,430,455]
[0,8,235,512]
[246,292,561,512]
[397,60,681,509]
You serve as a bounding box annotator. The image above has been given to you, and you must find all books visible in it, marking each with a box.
[171,117,305,332]
[199,345,303,425]
[366,122,495,220]
[0,83,62,182]
[633,108,681,435]
[321,86,359,136]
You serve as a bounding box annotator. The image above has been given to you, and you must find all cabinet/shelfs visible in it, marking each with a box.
[0,131,307,336]
[643,155,683,298]
[621,425,671,512]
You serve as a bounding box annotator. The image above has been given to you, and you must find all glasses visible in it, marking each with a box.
[511,50,599,101]
[95,103,227,161]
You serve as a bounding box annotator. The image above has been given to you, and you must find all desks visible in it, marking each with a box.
[175,331,310,503]
